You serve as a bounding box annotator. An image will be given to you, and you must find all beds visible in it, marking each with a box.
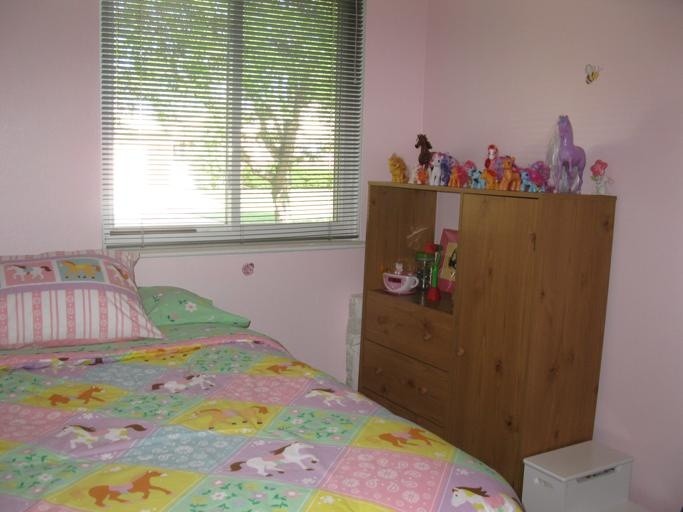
[0,287,519,511]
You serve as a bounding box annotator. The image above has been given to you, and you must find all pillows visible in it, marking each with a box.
[0,251,249,352]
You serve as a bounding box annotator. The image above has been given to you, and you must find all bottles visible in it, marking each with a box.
[415,252,434,290]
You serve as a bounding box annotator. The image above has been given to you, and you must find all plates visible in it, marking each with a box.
[380,287,416,296]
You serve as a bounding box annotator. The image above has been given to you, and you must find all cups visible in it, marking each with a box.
[382,271,419,292]
